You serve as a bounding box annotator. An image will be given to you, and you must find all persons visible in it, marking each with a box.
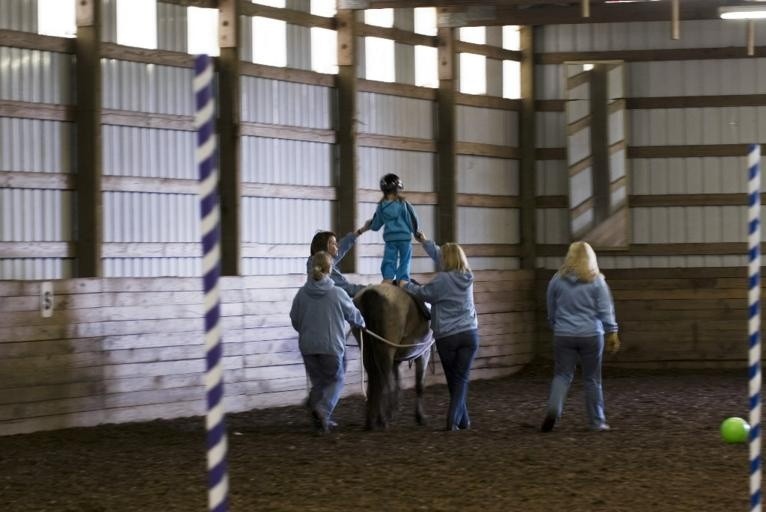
[290,250,368,436]
[400,233,478,432]
[307,220,373,373]
[367,174,421,284]
[541,241,620,433]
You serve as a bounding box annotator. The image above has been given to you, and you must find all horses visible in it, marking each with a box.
[350,277,436,431]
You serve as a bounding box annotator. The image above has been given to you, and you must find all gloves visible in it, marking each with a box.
[606,332,620,356]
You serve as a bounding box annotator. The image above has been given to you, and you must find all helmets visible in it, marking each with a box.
[380,173,403,193]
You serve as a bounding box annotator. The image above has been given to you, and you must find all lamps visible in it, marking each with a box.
[715,5,766,21]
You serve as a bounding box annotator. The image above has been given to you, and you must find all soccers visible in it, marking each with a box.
[720,416,749,445]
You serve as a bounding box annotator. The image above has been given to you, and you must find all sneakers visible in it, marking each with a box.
[540,413,556,433]
[312,404,338,437]
[445,418,471,432]
[591,423,612,432]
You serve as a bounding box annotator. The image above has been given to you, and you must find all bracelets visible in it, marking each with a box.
[358,230,362,235]
